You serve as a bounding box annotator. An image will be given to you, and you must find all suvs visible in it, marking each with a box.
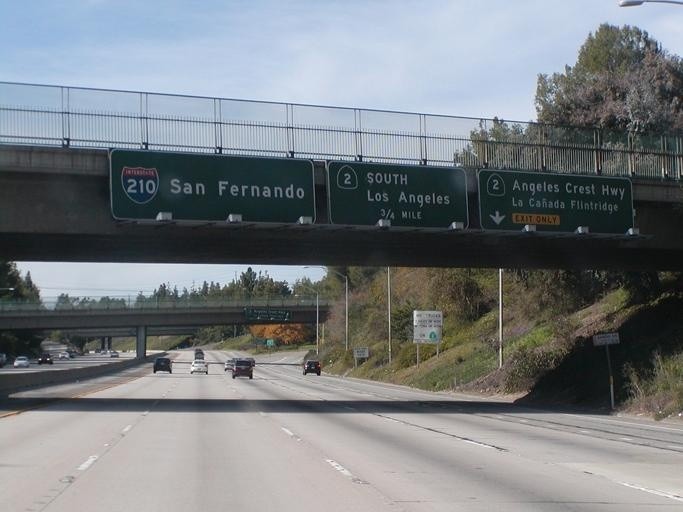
[303,360,321,376]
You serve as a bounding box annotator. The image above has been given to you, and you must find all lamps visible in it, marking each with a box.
[374,217,393,231]
[447,219,466,232]
[521,223,537,234]
[156,209,175,223]
[572,225,592,235]
[626,227,640,236]
[295,214,315,228]
[225,211,245,226]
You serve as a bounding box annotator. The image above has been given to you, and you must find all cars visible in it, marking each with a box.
[39,354,52,364]
[59,352,69,360]
[0,353,6,368]
[96,349,101,353]
[89,349,95,354]
[14,356,29,368]
[110,348,256,379]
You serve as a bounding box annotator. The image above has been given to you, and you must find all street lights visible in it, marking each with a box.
[619,0,683,7]
